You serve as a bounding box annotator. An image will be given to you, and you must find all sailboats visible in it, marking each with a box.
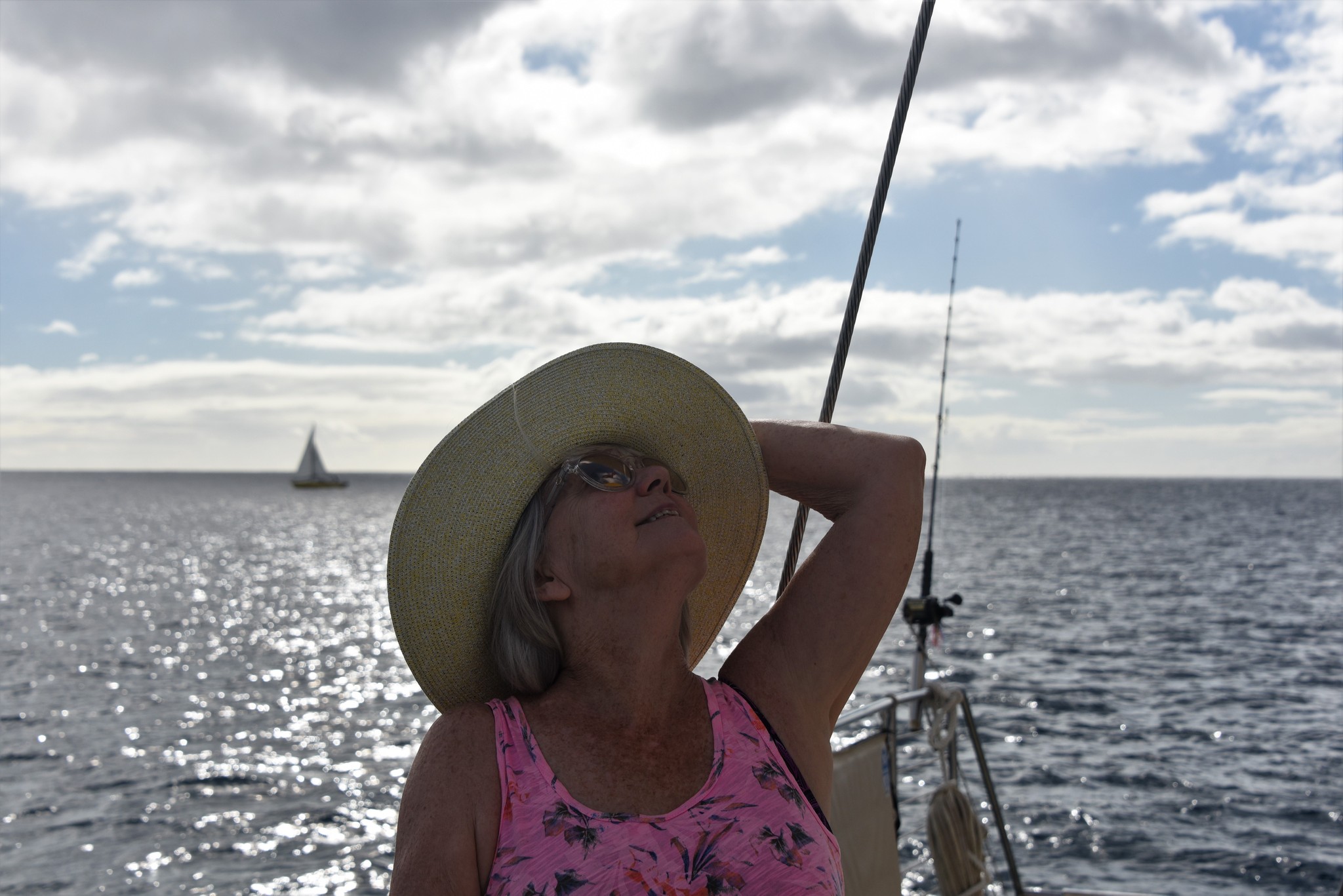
[291,425,348,489]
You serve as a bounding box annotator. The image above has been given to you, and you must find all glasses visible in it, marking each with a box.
[540,451,692,529]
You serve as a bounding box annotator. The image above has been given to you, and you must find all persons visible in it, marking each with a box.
[381,340,930,896]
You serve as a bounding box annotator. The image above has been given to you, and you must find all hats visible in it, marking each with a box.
[385,342,772,718]
